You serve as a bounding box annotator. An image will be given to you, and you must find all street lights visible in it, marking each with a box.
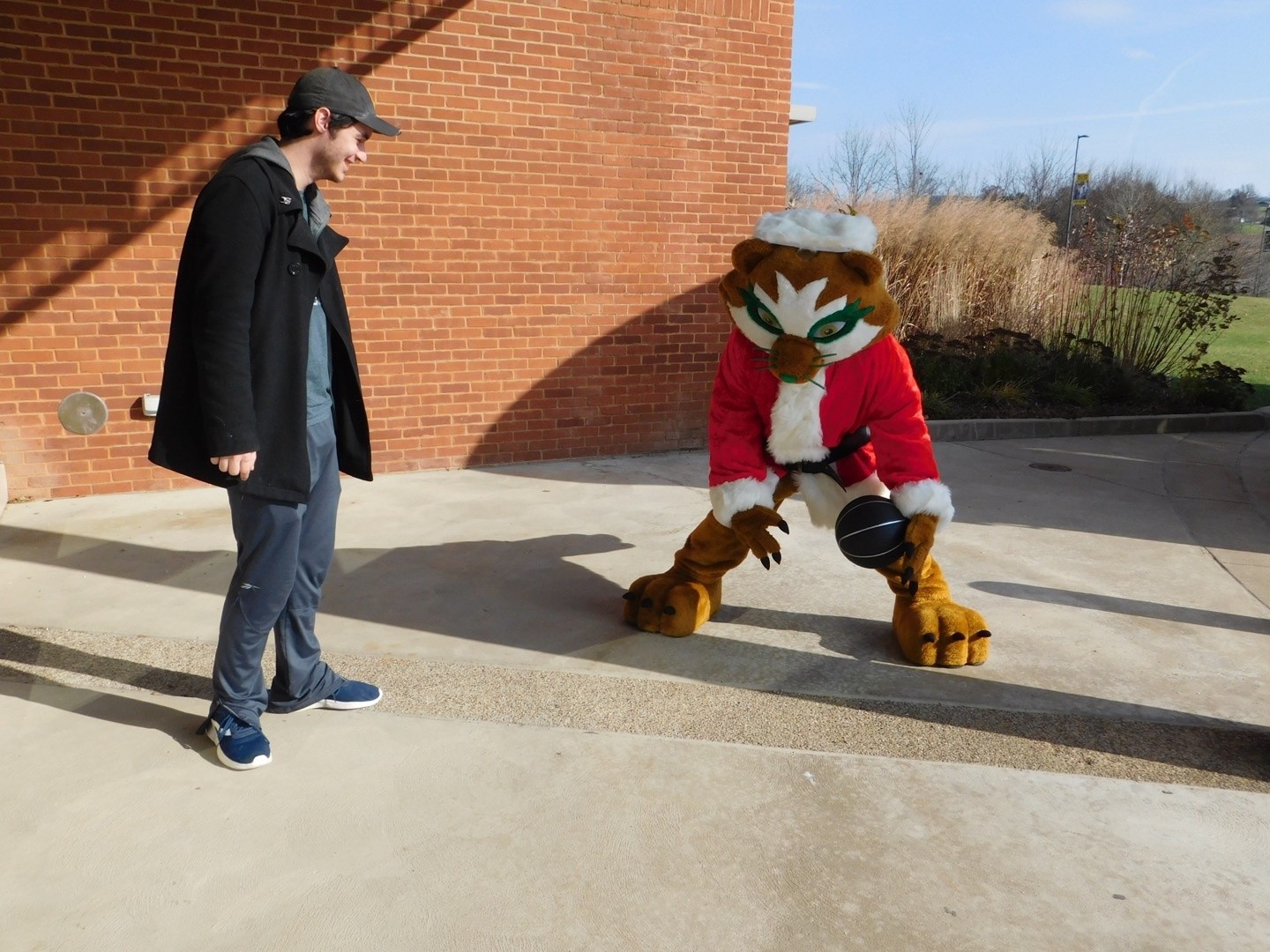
[1068,131,1089,246]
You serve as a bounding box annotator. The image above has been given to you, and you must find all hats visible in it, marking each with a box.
[288,65,401,136]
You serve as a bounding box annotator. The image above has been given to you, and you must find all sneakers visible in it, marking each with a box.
[205,704,273,770]
[288,673,382,712]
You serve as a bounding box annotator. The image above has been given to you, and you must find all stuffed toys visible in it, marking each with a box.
[622,208,994,670]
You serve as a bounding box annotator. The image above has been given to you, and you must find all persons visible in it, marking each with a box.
[144,66,402,770]
